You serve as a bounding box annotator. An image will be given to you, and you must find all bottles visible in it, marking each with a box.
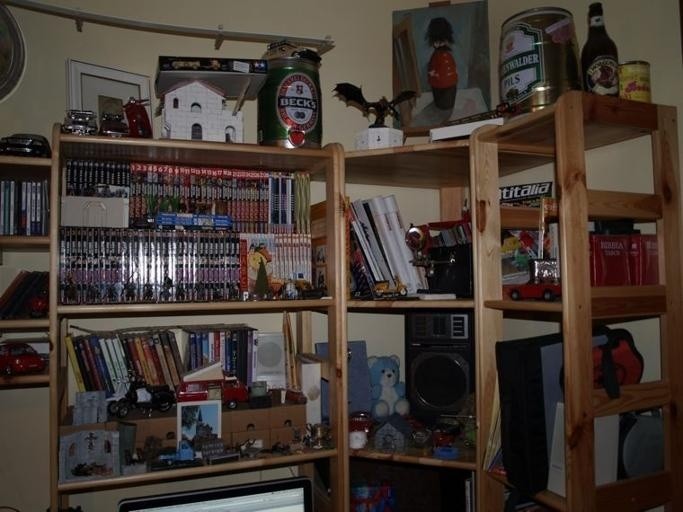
[580,5,619,98]
[499,8,580,122]
[257,59,320,151]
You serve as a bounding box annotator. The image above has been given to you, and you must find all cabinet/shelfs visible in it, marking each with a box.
[486,112,668,510]
[0,162,52,510]
[55,135,339,490]
[315,144,478,512]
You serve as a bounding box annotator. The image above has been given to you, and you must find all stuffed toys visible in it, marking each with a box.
[368,354,410,422]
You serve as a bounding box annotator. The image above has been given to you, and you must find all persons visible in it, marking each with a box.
[422,16,461,110]
[277,419,296,444]
[239,424,263,451]
[161,430,178,450]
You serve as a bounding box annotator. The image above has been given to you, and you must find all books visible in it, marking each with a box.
[549,221,660,286]
[5,270,48,320]
[55,322,259,407]
[58,421,120,483]
[5,179,51,237]
[316,338,372,424]
[345,194,428,300]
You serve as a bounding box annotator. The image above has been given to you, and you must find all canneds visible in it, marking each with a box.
[618,61,651,105]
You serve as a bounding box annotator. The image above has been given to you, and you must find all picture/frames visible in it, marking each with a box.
[177,399,222,461]
[65,57,154,138]
[241,232,276,294]
[55,429,120,487]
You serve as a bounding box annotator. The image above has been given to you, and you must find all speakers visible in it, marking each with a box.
[399,315,474,421]
